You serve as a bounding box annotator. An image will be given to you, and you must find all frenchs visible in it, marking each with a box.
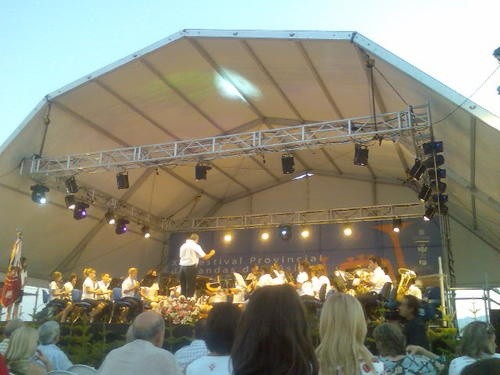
[395,266,418,303]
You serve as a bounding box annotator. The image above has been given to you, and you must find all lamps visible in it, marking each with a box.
[409,139,447,223]
[29,183,161,239]
[64,175,78,194]
[281,148,295,174]
[116,167,129,190]
[391,217,402,234]
[353,141,369,166]
[195,160,208,180]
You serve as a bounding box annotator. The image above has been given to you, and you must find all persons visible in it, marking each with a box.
[178,232,216,298]
[0,256,500,375]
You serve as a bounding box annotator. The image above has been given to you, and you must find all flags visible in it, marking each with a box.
[1,235,23,307]
